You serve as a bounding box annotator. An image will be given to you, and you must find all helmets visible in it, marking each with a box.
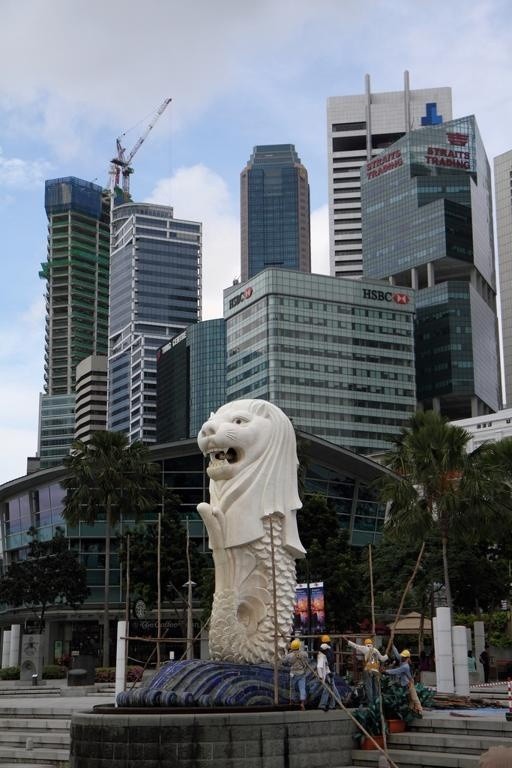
[291,635,410,658]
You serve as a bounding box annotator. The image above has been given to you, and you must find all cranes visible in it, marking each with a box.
[110,95,174,202]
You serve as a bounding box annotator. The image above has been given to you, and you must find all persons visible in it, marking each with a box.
[316,643,337,711]
[281,640,308,708]
[321,634,341,701]
[479,644,489,683]
[340,634,389,706]
[468,649,476,672]
[381,639,423,719]
